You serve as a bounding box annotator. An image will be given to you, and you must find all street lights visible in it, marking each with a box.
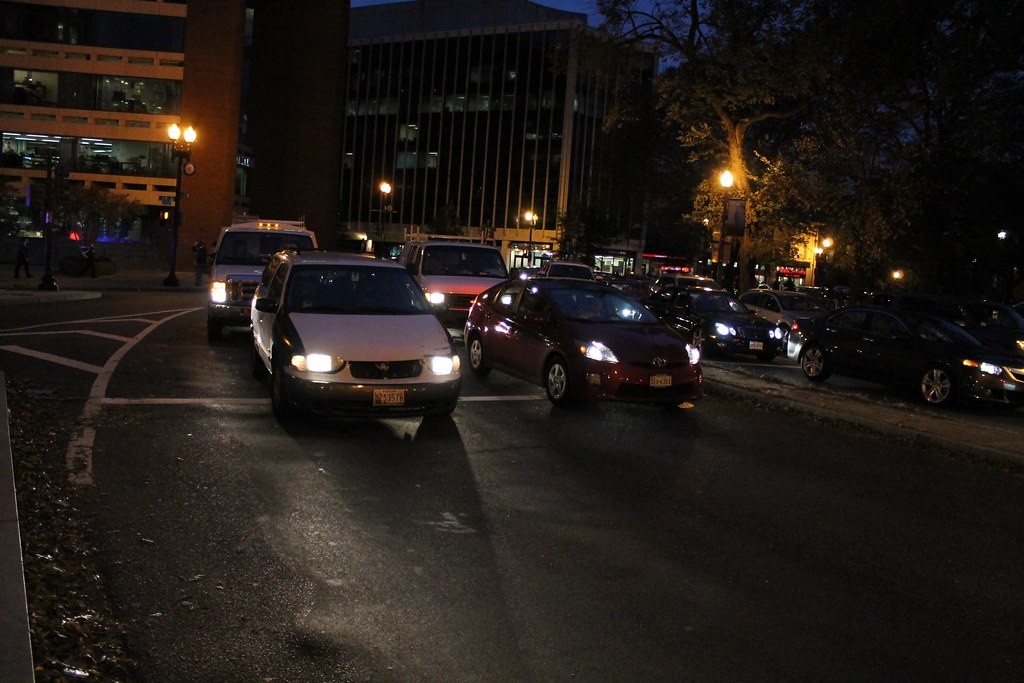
[719,171,747,296]
[34,145,61,291]
[377,182,392,257]
[160,123,199,286]
[523,210,538,269]
[814,239,832,303]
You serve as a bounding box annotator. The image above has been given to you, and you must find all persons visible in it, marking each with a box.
[13,237,34,279]
[192,239,207,286]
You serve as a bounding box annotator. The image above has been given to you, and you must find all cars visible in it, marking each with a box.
[463,276,703,411]
[544,262,1023,422]
[248,250,462,432]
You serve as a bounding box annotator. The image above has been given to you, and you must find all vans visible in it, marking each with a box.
[206,221,320,345]
[398,240,511,329]
